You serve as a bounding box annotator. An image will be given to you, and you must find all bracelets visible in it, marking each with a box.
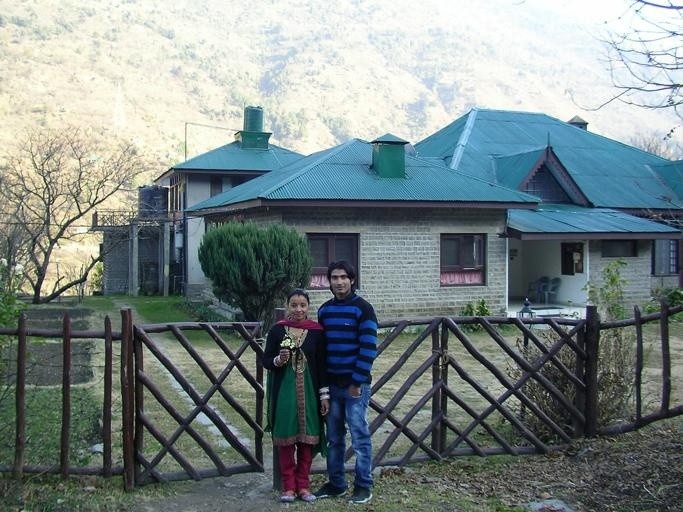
[318,385,331,401]
[273,355,287,369]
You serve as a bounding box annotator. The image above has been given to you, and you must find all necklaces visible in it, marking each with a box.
[287,323,306,345]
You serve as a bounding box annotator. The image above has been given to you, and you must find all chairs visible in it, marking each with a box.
[527,275,561,305]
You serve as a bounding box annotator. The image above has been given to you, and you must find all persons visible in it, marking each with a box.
[261,285,332,503]
[313,258,380,503]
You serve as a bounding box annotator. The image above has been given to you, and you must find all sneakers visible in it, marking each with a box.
[281,491,297,502]
[313,483,347,498]
[348,487,373,504]
[297,491,316,501]
